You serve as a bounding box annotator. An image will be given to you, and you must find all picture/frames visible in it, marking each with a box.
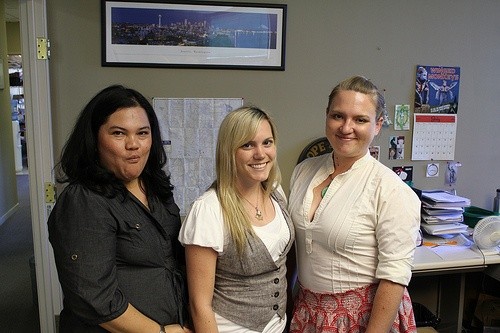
[100,0,288,71]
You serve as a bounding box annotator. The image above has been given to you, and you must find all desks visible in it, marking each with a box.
[411,228,500,333]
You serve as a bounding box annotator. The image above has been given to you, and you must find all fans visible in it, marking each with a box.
[472,216,500,249]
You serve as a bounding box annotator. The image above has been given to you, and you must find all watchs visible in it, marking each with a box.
[158,324,166,333]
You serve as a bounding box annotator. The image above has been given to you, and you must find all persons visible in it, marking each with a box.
[47,84,197,333]
[177,106,295,333]
[290,75,421,333]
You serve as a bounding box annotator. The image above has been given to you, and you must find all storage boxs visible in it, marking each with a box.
[463,206,500,228]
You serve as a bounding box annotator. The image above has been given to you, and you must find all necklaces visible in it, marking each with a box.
[235,185,266,221]
[318,176,333,198]
